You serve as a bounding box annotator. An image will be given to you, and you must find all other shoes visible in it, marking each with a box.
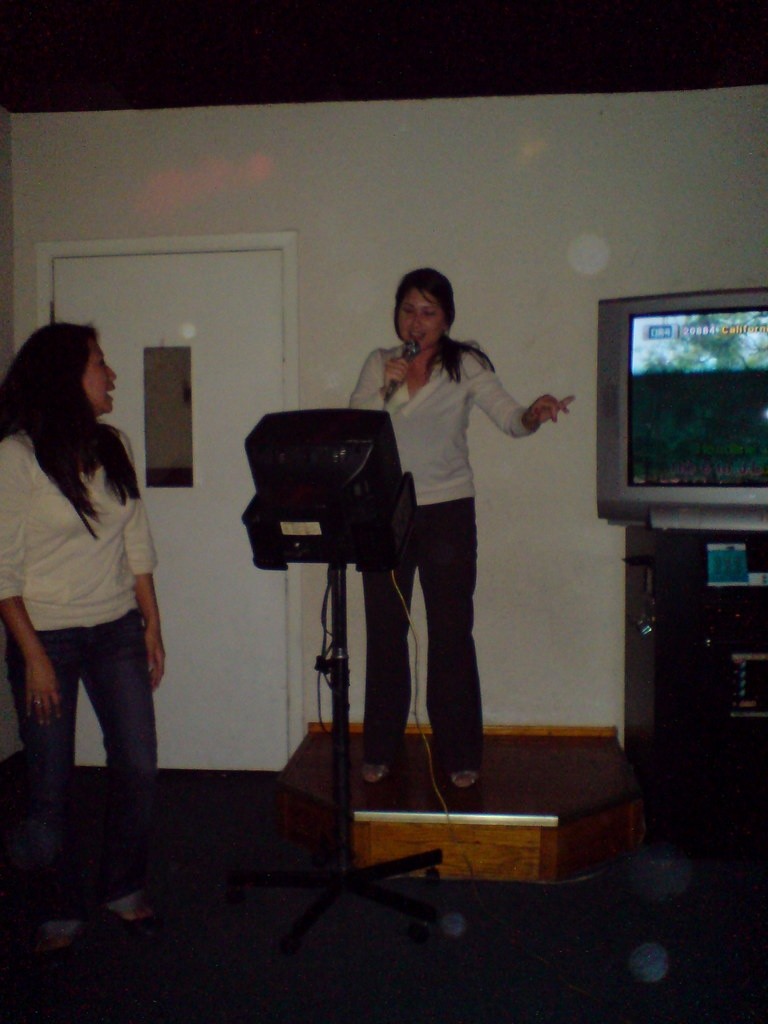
[104,891,151,922]
[41,919,86,953]
[451,769,477,788]
[362,764,387,783]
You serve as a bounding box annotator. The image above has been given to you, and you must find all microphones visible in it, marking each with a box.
[384,340,421,402]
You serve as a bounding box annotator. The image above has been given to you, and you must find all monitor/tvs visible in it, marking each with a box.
[596,287,768,530]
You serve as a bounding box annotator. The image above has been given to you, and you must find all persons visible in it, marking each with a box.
[349,268,576,788]
[0,323,166,951]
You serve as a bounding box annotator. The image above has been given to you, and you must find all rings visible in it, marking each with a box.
[34,700,42,704]
